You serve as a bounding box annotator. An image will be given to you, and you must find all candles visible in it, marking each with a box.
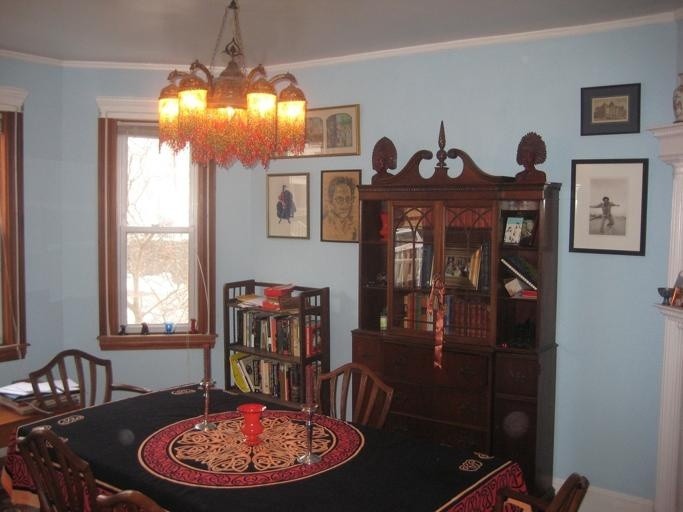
[204,344,211,381]
[306,364,314,407]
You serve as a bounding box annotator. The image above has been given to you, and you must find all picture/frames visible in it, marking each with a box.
[265,173,310,239]
[580,82,641,136]
[269,104,361,158]
[320,169,361,244]
[568,157,650,257]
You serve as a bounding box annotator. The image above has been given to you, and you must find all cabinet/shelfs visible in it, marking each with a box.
[223,279,331,417]
[350,120,562,503]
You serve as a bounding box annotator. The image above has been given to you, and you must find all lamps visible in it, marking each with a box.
[157,0,308,172]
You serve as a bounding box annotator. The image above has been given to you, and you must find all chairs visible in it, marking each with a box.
[16,427,96,512]
[320,363,394,431]
[497,471,590,512]
[95,490,170,512]
[29,349,151,417]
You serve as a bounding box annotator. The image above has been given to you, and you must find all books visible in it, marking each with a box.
[229,284,321,405]
[501,255,537,291]
[0,378,81,415]
[404,292,490,338]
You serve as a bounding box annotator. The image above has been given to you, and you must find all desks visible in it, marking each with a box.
[0,404,82,452]
[18,382,529,512]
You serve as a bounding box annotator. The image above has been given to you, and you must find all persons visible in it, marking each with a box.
[589,196,620,232]
[323,176,357,241]
